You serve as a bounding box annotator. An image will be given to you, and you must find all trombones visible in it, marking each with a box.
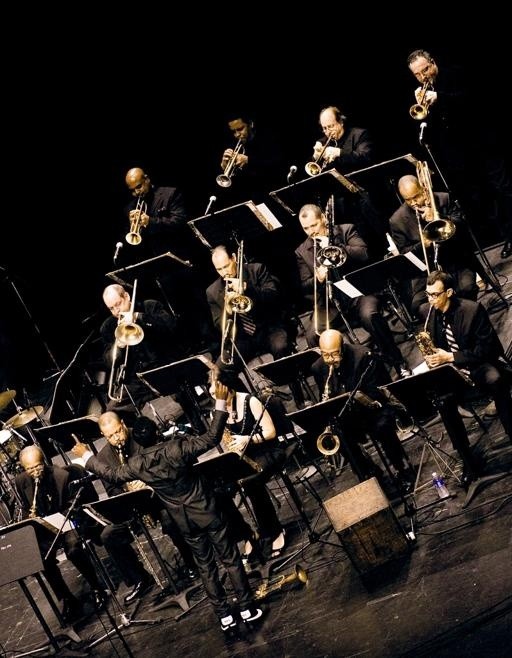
[220,241,255,366]
[413,160,457,275]
[311,195,349,335]
[107,278,145,403]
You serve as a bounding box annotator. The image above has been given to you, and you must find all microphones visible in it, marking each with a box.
[367,351,392,364]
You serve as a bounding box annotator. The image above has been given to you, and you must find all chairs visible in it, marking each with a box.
[26,201,512,615]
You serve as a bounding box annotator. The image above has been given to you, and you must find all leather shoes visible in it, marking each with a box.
[270,526,289,559]
[61,596,80,625]
[123,573,157,606]
[183,565,200,581]
[90,585,108,613]
[296,400,314,410]
[500,239,512,259]
[241,536,258,563]
[457,456,486,489]
[396,467,415,494]
[394,361,412,379]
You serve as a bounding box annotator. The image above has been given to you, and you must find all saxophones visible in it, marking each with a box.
[117,443,158,532]
[221,427,264,473]
[29,476,40,518]
[414,304,477,388]
[317,364,341,456]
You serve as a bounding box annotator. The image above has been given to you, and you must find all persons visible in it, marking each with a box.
[389,175,485,334]
[68,380,265,634]
[83,410,200,608]
[424,271,512,489]
[403,49,511,258]
[121,167,188,262]
[307,106,372,176]
[203,366,288,566]
[17,444,109,628]
[209,114,283,206]
[100,284,178,427]
[203,242,307,410]
[498,223,511,258]
[291,203,413,377]
[310,328,418,494]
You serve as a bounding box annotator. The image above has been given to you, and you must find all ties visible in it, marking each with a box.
[440,314,471,380]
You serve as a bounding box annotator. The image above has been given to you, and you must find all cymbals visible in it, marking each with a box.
[0,389,16,410]
[2,406,43,428]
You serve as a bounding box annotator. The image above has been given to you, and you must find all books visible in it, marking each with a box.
[187,451,261,487]
[79,487,158,528]
[343,153,435,195]
[281,392,381,436]
[381,362,468,415]
[186,200,284,251]
[132,355,218,400]
[251,345,327,388]
[330,250,427,305]
[29,412,106,460]
[267,167,369,218]
[104,251,195,303]
[0,510,76,540]
[0,518,61,544]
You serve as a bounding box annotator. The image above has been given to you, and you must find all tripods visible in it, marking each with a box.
[1,285,194,532]
[67,516,165,651]
[1,642,50,658]
[426,145,512,324]
[203,281,493,592]
[129,506,204,611]
[28,571,87,652]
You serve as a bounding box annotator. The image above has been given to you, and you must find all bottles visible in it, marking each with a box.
[431,472,449,499]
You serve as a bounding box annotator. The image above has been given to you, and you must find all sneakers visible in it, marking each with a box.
[219,614,240,641]
[239,603,270,634]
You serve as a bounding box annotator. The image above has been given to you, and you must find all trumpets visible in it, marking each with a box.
[215,139,246,188]
[408,79,436,122]
[233,563,308,600]
[304,134,333,177]
[125,193,147,247]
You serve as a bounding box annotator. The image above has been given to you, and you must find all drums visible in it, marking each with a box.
[0,429,25,467]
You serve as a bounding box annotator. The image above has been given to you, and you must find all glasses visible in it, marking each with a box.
[423,289,447,300]
